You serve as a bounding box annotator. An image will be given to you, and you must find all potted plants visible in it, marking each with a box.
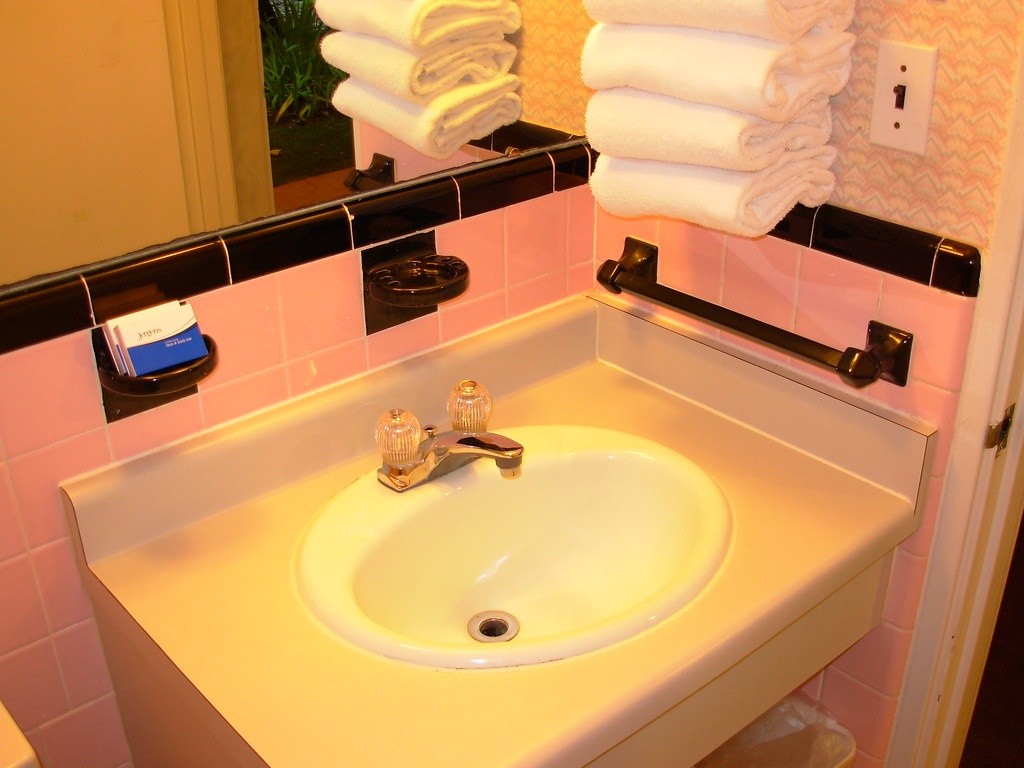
[257,0,351,187]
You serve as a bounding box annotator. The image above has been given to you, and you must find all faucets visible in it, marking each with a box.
[373,377,527,497]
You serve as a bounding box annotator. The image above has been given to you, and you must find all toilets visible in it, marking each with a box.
[1,697,42,768]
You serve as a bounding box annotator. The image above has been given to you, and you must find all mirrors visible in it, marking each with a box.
[0,0,596,293]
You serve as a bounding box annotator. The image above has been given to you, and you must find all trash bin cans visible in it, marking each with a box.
[693,695,858,768]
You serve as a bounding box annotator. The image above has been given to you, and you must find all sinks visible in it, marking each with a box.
[294,426,729,668]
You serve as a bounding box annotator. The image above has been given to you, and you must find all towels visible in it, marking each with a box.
[577,2,859,236]
[316,3,525,155]
